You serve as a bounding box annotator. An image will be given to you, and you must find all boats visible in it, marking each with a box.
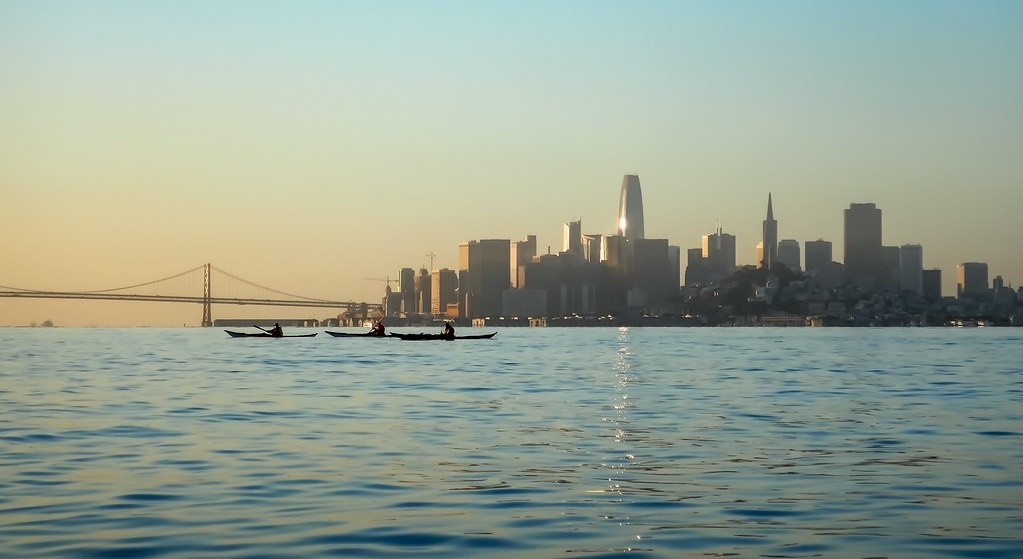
[224,329,317,338]
[325,330,424,337]
[390,331,498,341]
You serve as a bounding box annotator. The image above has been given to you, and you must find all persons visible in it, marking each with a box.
[266,322,283,337]
[371,320,385,335]
[444,322,455,338]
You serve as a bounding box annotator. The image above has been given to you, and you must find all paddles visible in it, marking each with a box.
[254,324,266,331]
[369,317,384,332]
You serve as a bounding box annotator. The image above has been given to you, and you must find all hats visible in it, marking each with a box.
[274,323,279,326]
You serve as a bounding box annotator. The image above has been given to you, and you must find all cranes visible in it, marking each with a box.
[424,251,435,270]
[365,276,398,286]
